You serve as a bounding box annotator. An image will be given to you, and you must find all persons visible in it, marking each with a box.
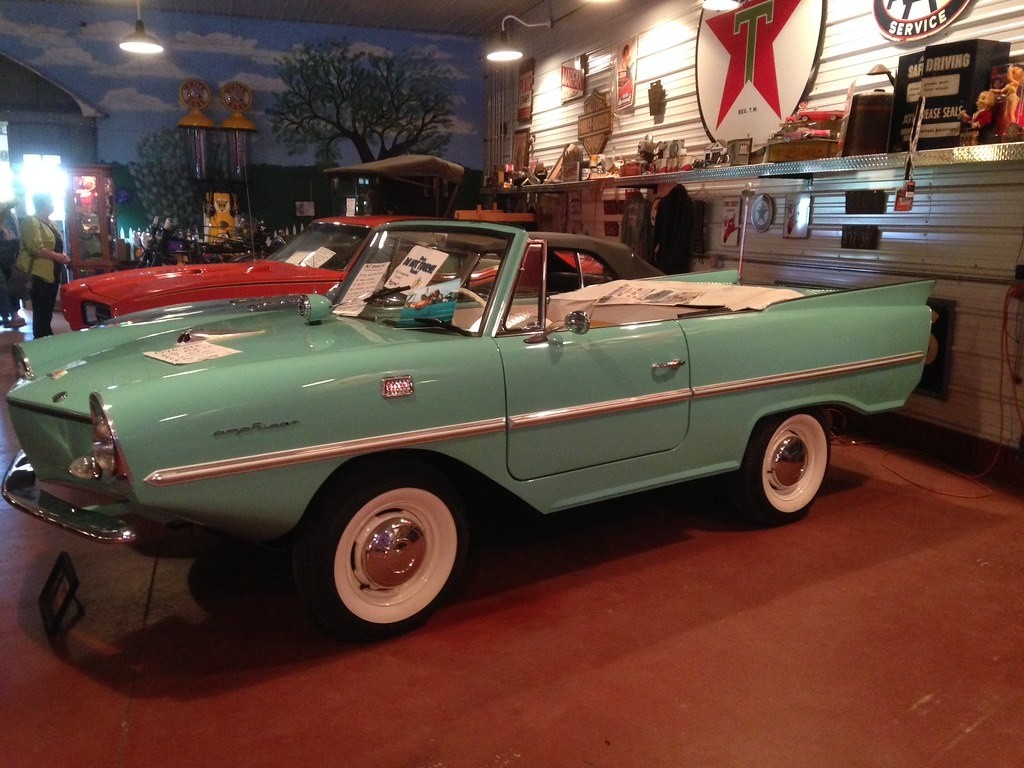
[0,178,72,340]
[989,64,1024,125]
[959,91,995,145]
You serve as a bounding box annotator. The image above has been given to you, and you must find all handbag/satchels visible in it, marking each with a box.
[0,267,36,300]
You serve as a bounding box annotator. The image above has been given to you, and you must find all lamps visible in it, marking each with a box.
[487,14,551,60]
[119,0,163,53]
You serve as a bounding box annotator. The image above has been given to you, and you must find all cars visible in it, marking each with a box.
[58,213,639,336]
[0,217,936,642]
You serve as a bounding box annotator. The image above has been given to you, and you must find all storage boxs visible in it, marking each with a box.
[888,39,1010,152]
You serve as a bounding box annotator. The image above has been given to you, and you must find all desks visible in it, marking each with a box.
[479,128,1024,193]
